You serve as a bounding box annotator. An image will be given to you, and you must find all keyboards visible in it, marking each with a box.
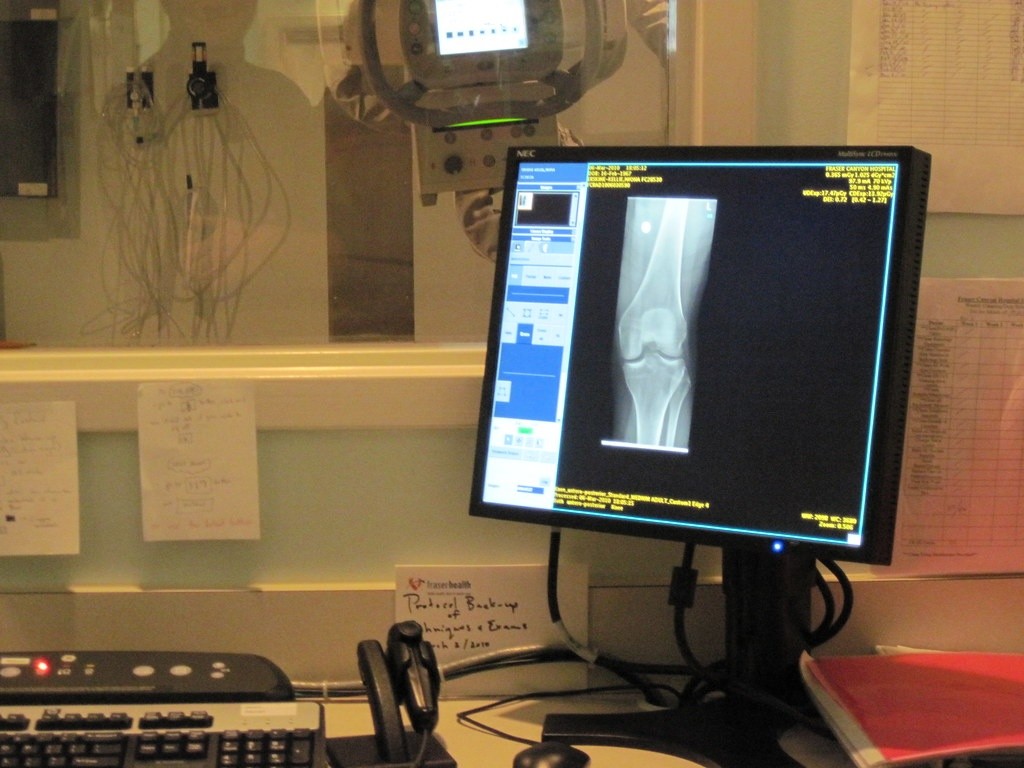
[0,702,326,768]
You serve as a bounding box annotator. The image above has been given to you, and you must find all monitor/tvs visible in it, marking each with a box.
[434,0,529,56]
[466,146,933,768]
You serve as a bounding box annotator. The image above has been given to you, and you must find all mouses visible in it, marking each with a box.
[513,742,589,768]
